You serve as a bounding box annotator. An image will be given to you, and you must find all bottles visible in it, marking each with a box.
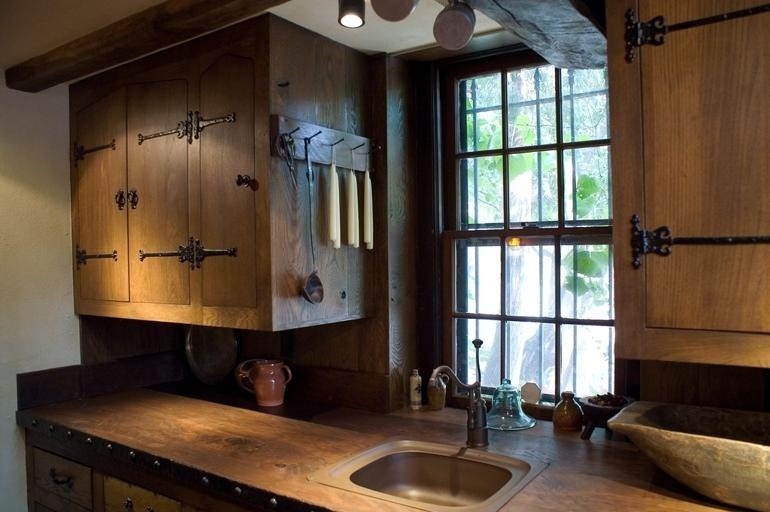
[551,391,584,433]
[410,369,423,411]
[429,373,449,411]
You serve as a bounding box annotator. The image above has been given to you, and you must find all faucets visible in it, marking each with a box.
[429,339,489,447]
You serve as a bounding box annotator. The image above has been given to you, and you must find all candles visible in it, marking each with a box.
[329,163,374,250]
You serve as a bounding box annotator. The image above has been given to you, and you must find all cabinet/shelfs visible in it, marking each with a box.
[94,473,222,512]
[23,429,92,511]
[189,15,368,332]
[605,1,768,369]
[70,41,189,332]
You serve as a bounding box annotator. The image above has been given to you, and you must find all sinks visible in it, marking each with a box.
[306,434,550,511]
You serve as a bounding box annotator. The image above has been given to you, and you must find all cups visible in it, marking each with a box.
[246,359,292,408]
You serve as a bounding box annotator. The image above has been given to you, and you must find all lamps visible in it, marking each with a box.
[339,1,476,52]
